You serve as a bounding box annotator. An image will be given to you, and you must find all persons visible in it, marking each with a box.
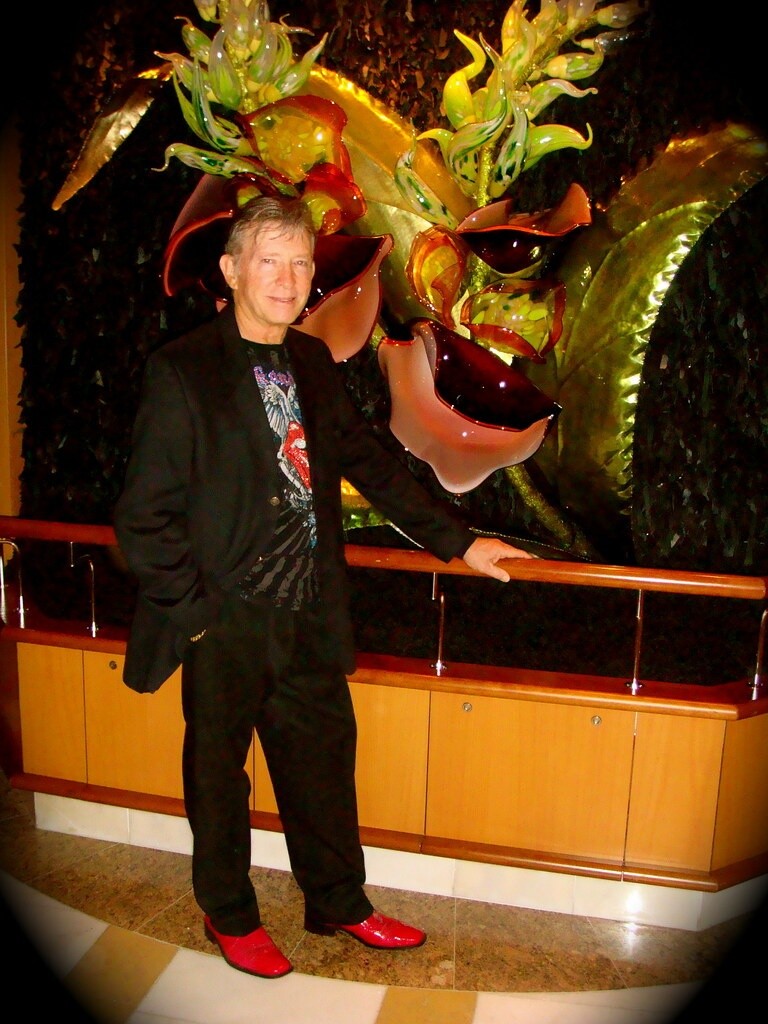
[110,192,534,978]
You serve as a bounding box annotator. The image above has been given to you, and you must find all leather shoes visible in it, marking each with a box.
[203,914,294,978]
[304,908,426,949]
